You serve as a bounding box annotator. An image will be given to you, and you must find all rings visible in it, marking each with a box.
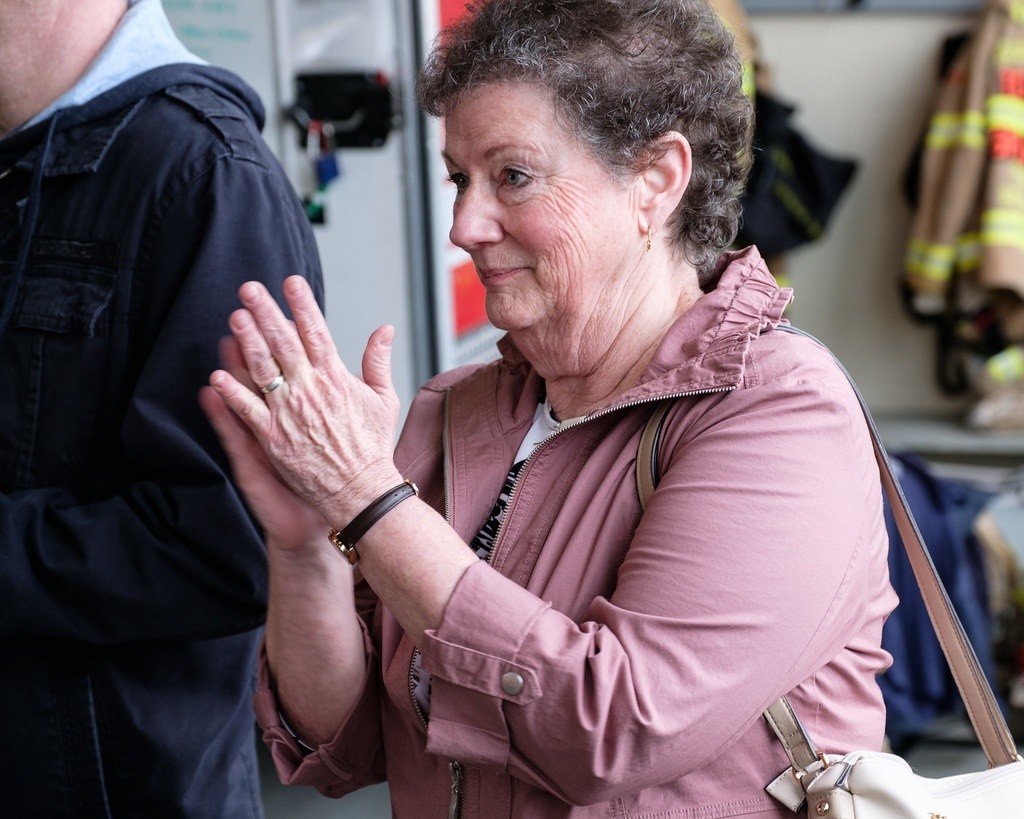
[260,375,285,394]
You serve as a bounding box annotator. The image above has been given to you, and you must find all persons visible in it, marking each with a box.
[0,0,325,819]
[201,0,899,819]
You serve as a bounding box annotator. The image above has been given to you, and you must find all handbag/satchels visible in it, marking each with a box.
[764,750,1024,819]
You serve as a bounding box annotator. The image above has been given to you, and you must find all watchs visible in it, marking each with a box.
[328,479,419,566]
